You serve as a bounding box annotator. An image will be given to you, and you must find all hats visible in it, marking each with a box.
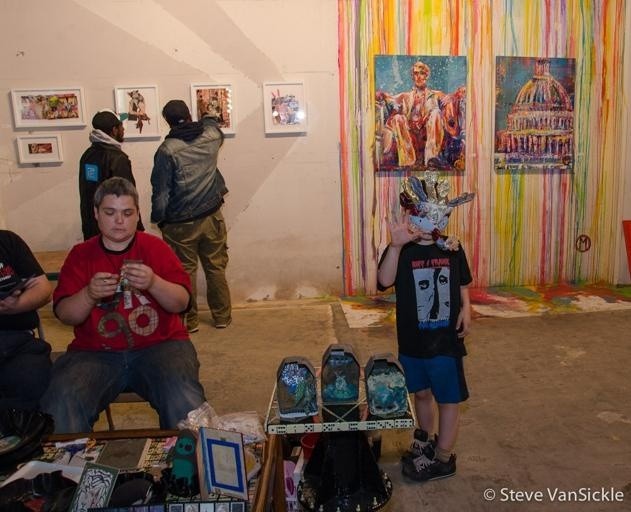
[162,99,189,125]
[91,108,127,130]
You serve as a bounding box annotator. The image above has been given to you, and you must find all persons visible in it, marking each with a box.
[150,98,235,334]
[413,268,434,322]
[49,176,209,438]
[435,266,451,321]
[77,109,148,240]
[375,177,476,483]
[376,60,455,168]
[0,229,55,430]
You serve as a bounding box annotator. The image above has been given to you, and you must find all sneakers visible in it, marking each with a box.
[399,428,438,465]
[215,316,231,328]
[401,445,456,483]
